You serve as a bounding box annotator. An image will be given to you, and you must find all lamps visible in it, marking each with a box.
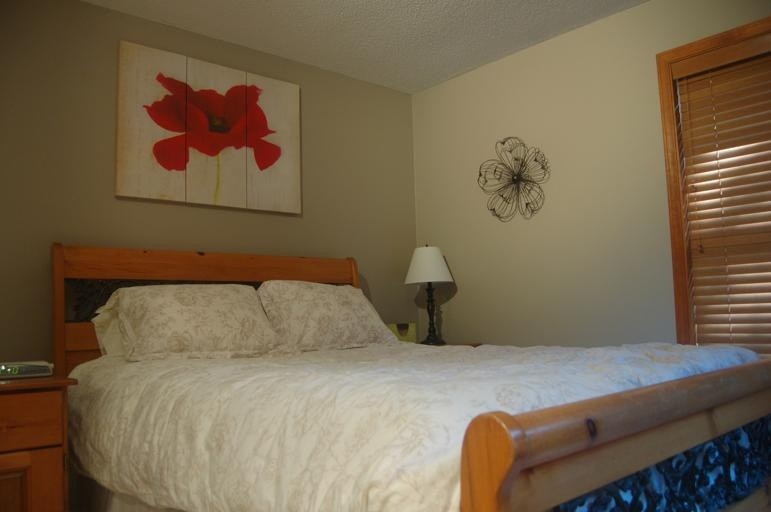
[404,241,457,347]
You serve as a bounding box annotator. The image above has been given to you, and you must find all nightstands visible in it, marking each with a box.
[2,372,80,512]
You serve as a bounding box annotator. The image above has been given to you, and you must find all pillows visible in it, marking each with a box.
[92,306,125,356]
[106,284,276,362]
[258,280,400,352]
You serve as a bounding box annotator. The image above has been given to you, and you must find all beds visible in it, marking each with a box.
[46,240,771,510]
